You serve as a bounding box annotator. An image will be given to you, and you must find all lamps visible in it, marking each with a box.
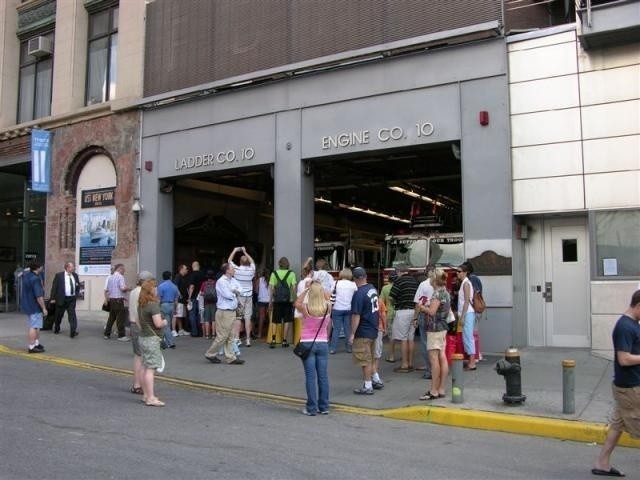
[375,213,389,219]
[348,207,363,212]
[403,191,421,198]
[389,216,400,221]
[432,200,436,204]
[389,186,406,194]
[362,210,376,216]
[339,203,349,209]
[400,219,411,223]
[314,196,333,204]
[420,195,432,203]
[436,200,444,206]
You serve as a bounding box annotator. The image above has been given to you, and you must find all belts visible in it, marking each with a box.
[223,309,237,311]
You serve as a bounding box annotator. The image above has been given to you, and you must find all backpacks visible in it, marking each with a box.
[463,280,487,313]
[272,270,292,304]
[205,282,217,304]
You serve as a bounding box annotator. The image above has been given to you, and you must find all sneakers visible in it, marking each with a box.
[205,336,211,339]
[330,350,336,354]
[103,336,111,340]
[423,373,432,379]
[147,397,166,406]
[171,330,178,337]
[269,342,276,348]
[346,348,352,353]
[406,365,415,372]
[354,386,373,395]
[303,407,317,416]
[117,336,132,341]
[372,381,384,389]
[394,366,409,373]
[212,335,216,339]
[140,396,159,403]
[245,339,251,347]
[282,344,289,348]
[178,329,191,336]
[413,365,427,371]
[236,339,241,347]
[317,407,330,414]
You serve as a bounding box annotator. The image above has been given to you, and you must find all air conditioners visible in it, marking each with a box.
[27,36,53,58]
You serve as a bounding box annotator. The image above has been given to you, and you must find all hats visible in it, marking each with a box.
[353,267,367,279]
[138,271,157,283]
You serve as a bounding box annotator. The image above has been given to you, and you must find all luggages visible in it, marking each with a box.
[40,297,55,331]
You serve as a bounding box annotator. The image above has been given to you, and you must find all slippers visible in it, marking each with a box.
[437,393,445,398]
[462,365,477,371]
[591,465,627,477]
[419,391,438,400]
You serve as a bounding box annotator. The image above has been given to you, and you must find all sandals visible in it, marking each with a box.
[131,384,144,394]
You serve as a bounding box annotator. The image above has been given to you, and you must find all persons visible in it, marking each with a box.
[379,262,419,373]
[254,257,297,349]
[416,262,484,400]
[156,261,203,349]
[129,271,168,406]
[592,290,640,477]
[296,258,335,414]
[104,264,132,342]
[332,266,385,394]
[19,261,48,353]
[201,246,256,365]
[104,265,119,335]
[50,262,80,338]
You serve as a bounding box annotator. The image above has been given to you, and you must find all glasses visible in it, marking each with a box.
[457,270,463,273]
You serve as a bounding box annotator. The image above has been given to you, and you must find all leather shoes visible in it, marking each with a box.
[70,332,79,338]
[229,359,245,364]
[54,327,60,334]
[29,345,44,353]
[205,356,221,363]
[37,343,45,349]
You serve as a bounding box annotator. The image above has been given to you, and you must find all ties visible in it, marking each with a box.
[69,274,74,294]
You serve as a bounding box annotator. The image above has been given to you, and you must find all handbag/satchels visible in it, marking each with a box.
[155,353,165,373]
[102,300,112,312]
[293,342,311,360]
[329,288,336,306]
[445,306,456,323]
